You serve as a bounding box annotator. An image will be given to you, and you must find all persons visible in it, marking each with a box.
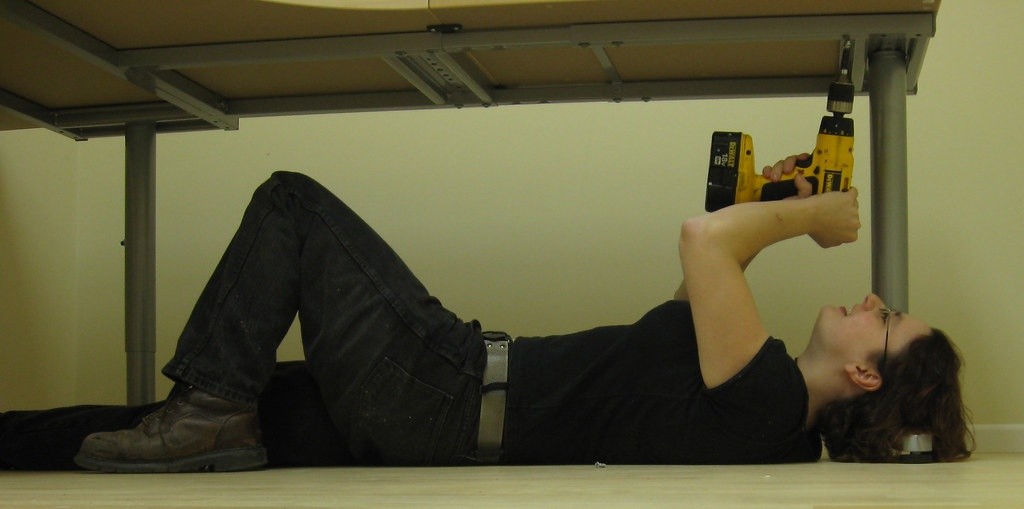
[75,153,976,474]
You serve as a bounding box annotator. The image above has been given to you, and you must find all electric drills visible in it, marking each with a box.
[705,40,856,212]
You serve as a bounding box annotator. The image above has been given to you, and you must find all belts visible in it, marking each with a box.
[477,340,509,465]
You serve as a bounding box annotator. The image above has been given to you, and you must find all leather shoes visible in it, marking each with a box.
[74,385,267,472]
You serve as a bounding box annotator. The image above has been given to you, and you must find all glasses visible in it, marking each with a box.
[878,306,896,374]
